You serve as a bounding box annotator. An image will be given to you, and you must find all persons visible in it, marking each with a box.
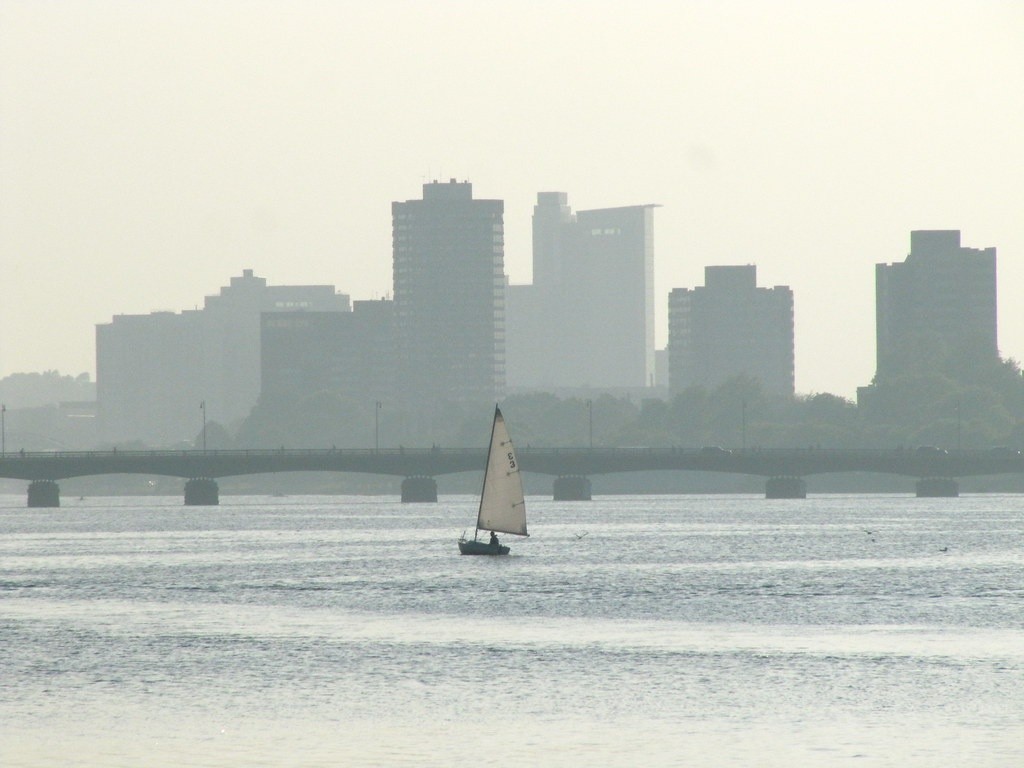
[490,532,499,545]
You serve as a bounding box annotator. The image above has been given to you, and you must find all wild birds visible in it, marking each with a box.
[940,547,948,551]
[864,530,879,535]
[575,532,588,540]
[872,539,876,542]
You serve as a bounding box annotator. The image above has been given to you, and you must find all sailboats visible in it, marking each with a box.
[459,404,530,556]
